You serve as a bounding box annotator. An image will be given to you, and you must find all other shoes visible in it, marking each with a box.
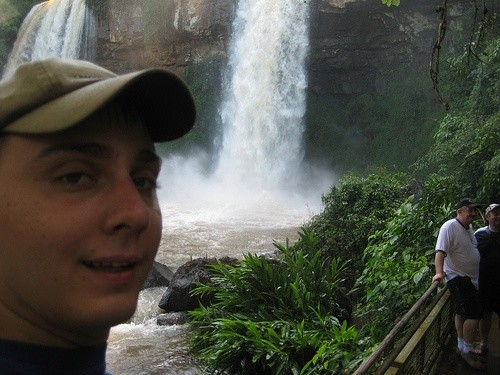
[460,348,484,370]
[480,344,488,361]
[456,347,463,357]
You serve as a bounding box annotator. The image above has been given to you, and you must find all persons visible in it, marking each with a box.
[474,203,500,355]
[431,198,481,354]
[0,57,197,375]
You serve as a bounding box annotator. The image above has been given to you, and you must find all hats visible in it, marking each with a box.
[0,56,198,156]
[455,198,482,210]
[485,203,500,214]
